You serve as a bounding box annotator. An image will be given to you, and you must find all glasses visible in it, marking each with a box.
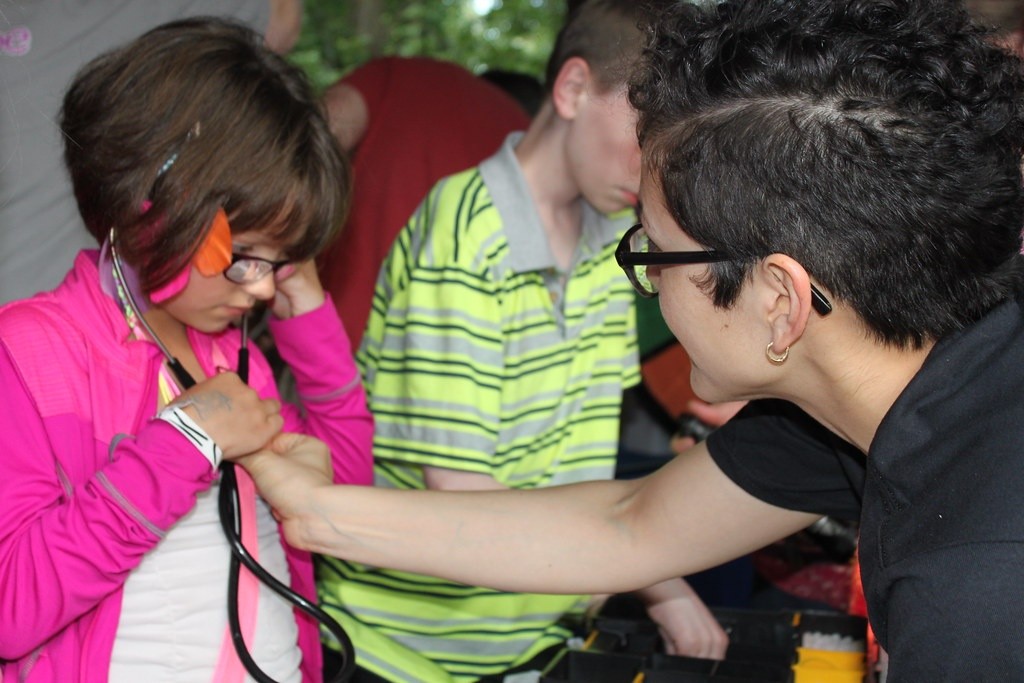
[222,252,290,285]
[615,224,832,317]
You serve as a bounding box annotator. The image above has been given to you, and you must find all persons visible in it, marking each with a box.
[229,0,1024,683]
[316,0,728,683]
[320,54,541,355]
[0,14,373,683]
